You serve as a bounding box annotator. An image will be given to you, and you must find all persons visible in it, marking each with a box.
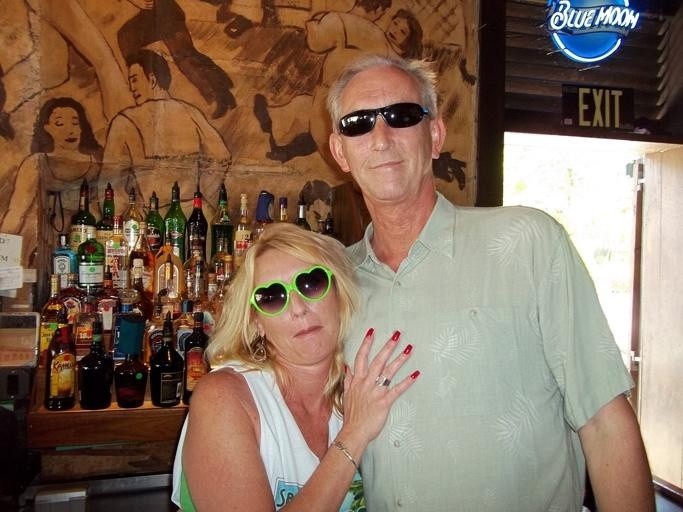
[172,219,421,511]
[328,53,658,510]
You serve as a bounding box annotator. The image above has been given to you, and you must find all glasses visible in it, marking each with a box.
[339,103,428,136]
[249,266,332,316]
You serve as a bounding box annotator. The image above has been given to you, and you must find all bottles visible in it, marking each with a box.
[39,175,333,409]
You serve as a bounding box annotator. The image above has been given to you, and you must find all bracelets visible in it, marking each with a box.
[329,435,361,472]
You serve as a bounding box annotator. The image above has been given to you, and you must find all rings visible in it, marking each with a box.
[374,373,391,386]
